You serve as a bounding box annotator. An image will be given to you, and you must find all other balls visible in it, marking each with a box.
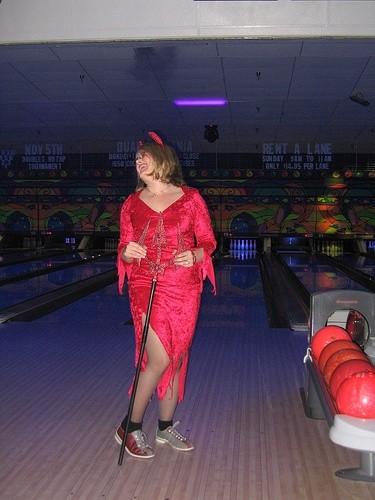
[312,324,352,360]
[317,337,362,375]
[323,348,369,386]
[336,371,375,418]
[328,360,374,399]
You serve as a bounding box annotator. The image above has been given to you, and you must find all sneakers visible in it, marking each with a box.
[156,421,194,451]
[113,425,155,458]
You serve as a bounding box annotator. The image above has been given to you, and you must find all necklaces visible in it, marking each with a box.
[146,183,172,199]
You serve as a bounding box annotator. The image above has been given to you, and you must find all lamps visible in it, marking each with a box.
[173,98,227,106]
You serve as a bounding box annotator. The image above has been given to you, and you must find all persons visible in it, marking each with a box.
[114,129,219,460]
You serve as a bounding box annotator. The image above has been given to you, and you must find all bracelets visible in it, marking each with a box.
[192,250,198,265]
